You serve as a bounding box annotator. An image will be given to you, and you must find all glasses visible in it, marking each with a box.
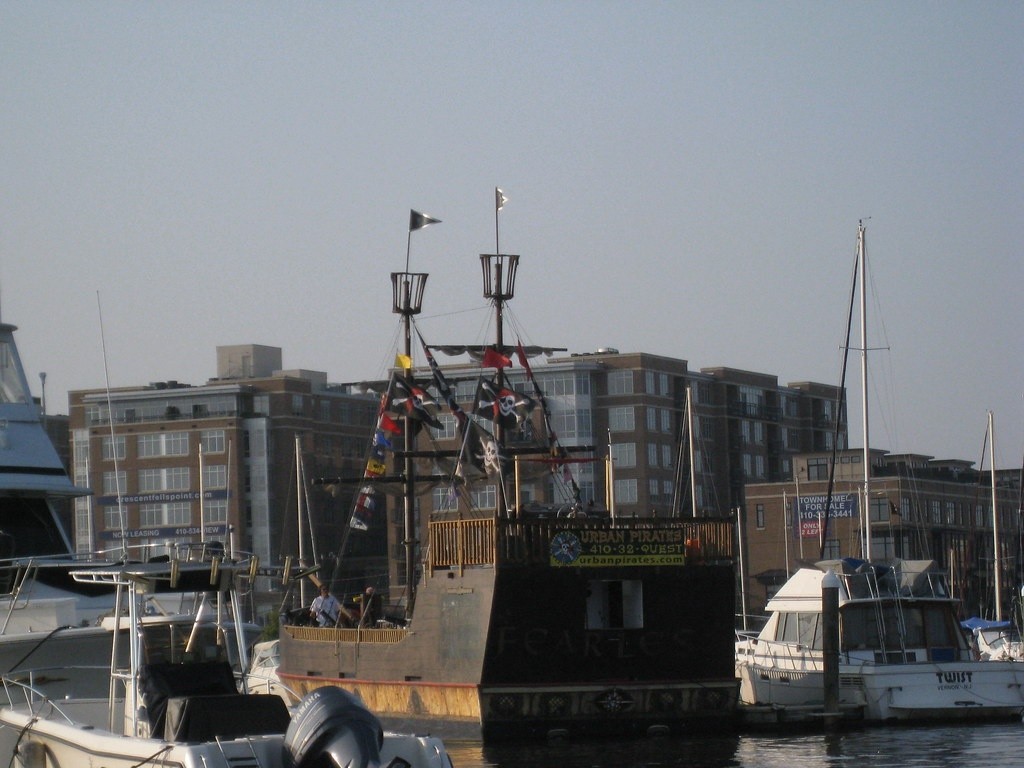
[320,589,329,591]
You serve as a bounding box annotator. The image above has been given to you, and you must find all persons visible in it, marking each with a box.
[308,579,383,628]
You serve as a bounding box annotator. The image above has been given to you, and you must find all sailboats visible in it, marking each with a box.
[734,215,1024,727]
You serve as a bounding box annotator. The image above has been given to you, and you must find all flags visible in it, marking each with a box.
[346,348,536,532]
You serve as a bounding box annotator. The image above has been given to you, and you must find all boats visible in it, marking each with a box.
[274,191,743,749]
[0,288,455,767]
